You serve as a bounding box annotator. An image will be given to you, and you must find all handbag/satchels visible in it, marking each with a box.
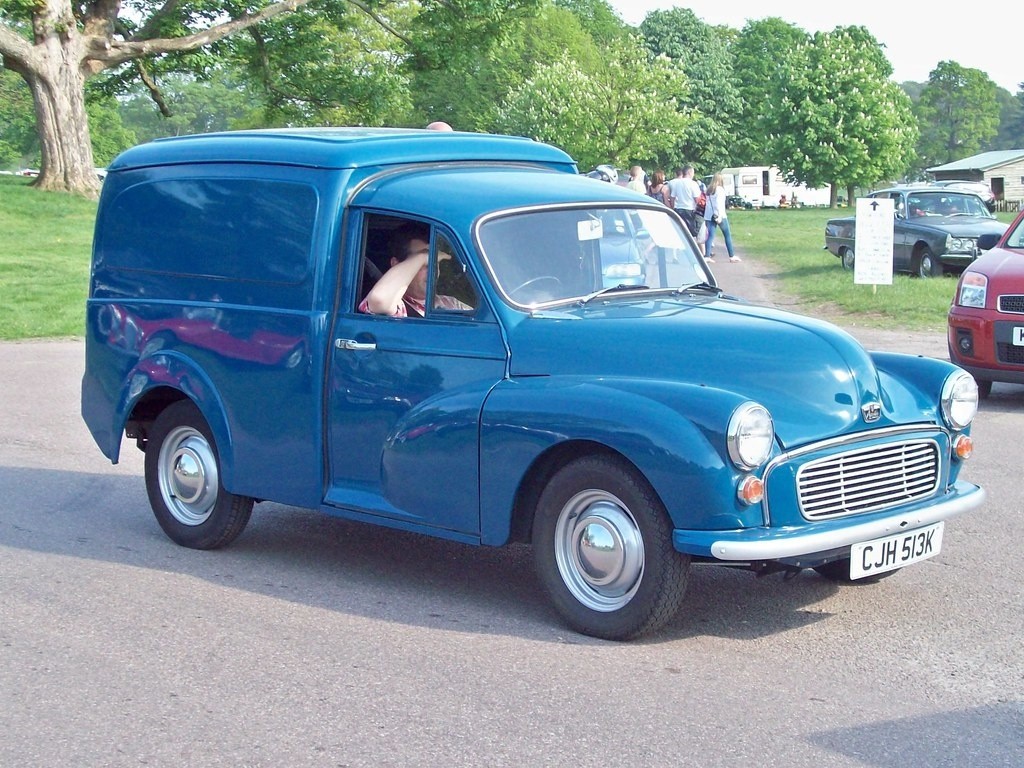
[711,214,721,224]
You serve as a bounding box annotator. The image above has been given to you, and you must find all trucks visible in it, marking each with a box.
[82,121,987,642]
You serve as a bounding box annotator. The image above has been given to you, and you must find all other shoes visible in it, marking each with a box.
[704,257,715,264]
[729,256,742,262]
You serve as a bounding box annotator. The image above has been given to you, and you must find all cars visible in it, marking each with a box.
[597,208,651,290]
[930,180,996,211]
[947,210,1024,399]
[824,188,1018,278]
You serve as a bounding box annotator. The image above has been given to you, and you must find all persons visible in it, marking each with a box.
[703,173,742,263]
[625,165,715,257]
[358,223,475,318]
